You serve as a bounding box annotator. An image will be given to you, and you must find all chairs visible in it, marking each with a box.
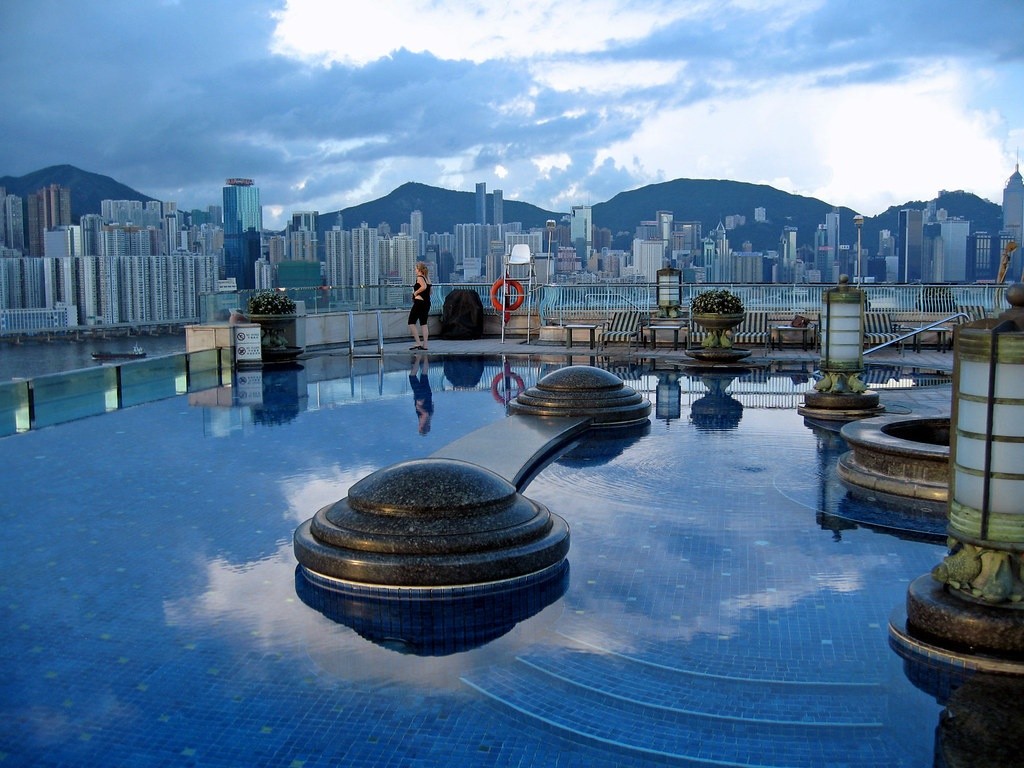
[735,311,772,358]
[597,310,643,355]
[687,307,715,350]
[813,313,822,352]
[863,313,905,354]
[956,305,987,330]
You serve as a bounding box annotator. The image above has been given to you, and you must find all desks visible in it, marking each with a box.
[911,327,952,354]
[564,324,598,347]
[649,324,684,351]
[775,326,811,351]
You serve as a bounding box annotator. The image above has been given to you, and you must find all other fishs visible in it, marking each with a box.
[700,331,732,348]
[849,374,867,393]
[930,542,1023,604]
[812,373,844,392]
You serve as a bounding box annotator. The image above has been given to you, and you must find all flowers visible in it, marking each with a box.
[246,288,298,316]
[691,290,745,315]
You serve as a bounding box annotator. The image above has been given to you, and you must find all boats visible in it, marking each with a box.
[90,341,147,359]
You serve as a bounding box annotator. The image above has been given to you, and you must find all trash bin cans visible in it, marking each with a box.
[285,300,307,351]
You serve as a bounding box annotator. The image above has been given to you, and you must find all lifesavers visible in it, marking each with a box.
[491,371,525,404]
[491,278,524,311]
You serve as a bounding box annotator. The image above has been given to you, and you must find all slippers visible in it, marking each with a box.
[408,344,420,350]
[418,346,428,350]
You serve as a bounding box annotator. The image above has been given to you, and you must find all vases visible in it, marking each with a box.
[242,313,305,351]
[692,313,745,348]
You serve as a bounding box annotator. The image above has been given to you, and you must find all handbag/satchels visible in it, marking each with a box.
[789,315,810,327]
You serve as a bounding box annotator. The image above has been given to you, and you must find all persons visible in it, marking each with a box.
[408,262,433,350]
[409,354,434,436]
